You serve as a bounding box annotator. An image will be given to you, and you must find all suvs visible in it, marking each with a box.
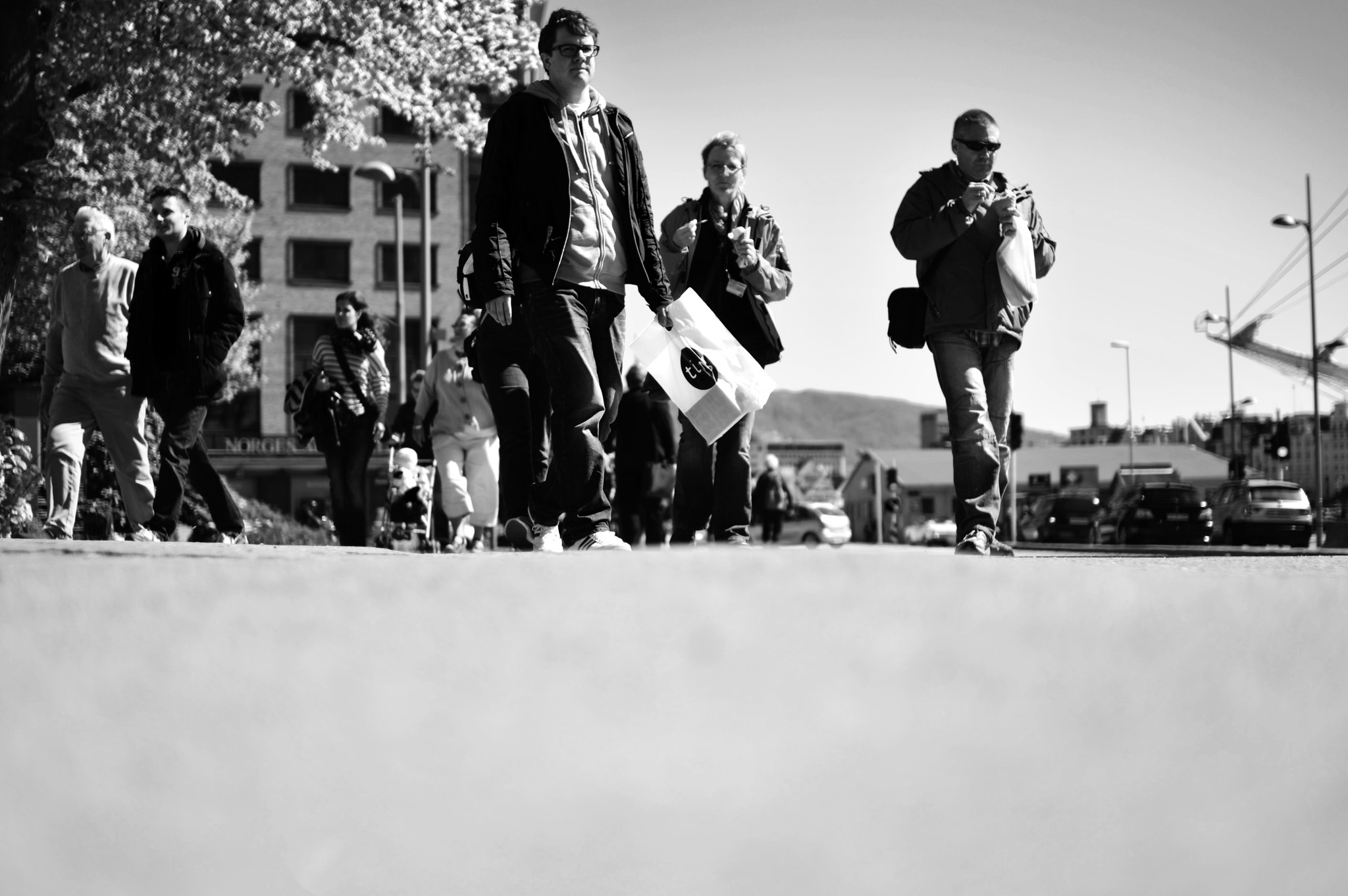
[1092,481,1215,547]
[1204,479,1313,548]
[1017,492,1102,545]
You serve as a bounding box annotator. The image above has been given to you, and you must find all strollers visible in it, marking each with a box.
[376,445,439,552]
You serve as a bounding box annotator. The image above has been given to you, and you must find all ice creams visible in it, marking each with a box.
[992,189,1017,218]
[727,226,751,258]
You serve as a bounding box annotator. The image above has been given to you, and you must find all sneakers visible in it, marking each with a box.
[532,518,563,552]
[992,539,1015,557]
[564,530,632,551]
[955,529,991,557]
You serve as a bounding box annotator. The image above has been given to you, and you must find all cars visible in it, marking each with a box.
[749,501,852,546]
[903,517,956,547]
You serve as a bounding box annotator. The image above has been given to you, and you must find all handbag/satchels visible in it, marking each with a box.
[629,287,776,446]
[457,242,483,307]
[887,287,926,353]
[996,215,1039,311]
[365,396,392,443]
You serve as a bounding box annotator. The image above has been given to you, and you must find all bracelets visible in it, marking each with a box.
[413,425,422,429]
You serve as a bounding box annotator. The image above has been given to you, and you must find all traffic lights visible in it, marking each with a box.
[1271,431,1291,460]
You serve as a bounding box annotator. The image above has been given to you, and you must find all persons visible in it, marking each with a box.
[751,453,795,543]
[388,369,451,553]
[283,290,391,548]
[887,109,1057,558]
[471,6,677,551]
[604,366,677,547]
[388,447,429,541]
[411,309,500,555]
[123,187,247,544]
[657,131,792,546]
[10,206,155,540]
[456,241,564,552]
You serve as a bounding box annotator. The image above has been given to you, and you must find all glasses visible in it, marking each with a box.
[549,43,600,57]
[411,386,420,394]
[70,228,107,237]
[954,137,1001,152]
[452,323,476,329]
[705,162,745,171]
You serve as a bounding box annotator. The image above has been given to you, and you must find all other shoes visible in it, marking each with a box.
[216,531,248,545]
[133,523,163,542]
[18,525,70,540]
[471,538,485,553]
[392,526,412,540]
[445,534,468,553]
[503,518,534,551]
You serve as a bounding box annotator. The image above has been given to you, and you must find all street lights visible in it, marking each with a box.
[350,160,407,408]
[1110,341,1136,484]
[1270,173,1324,547]
[1205,285,1241,478]
